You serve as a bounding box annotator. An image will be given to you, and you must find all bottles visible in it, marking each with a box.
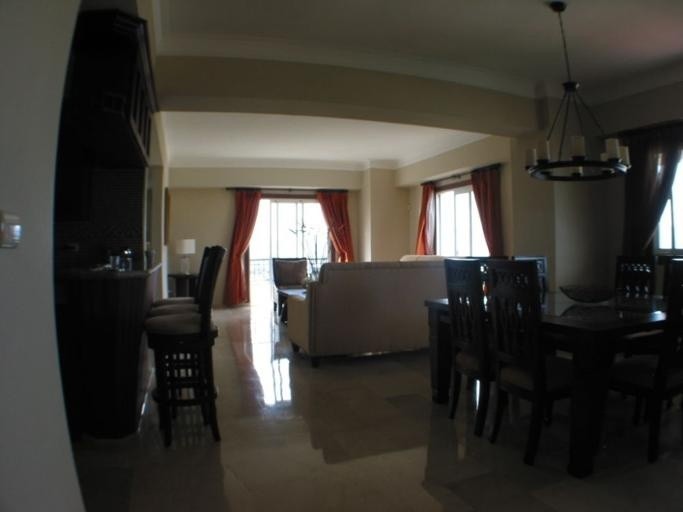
[121,257,133,273]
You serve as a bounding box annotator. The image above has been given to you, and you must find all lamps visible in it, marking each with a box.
[175,238,196,274]
[524,1,633,181]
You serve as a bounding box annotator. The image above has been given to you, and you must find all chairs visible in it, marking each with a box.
[145,244,226,447]
[443,252,683,466]
[272,258,308,315]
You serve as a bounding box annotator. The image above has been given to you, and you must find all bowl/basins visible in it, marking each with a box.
[559,284,626,303]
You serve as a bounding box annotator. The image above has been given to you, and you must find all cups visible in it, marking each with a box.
[110,255,120,271]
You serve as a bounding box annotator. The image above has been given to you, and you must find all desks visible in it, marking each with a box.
[423,290,669,478]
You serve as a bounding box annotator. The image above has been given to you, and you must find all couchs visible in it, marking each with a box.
[285,253,449,367]
